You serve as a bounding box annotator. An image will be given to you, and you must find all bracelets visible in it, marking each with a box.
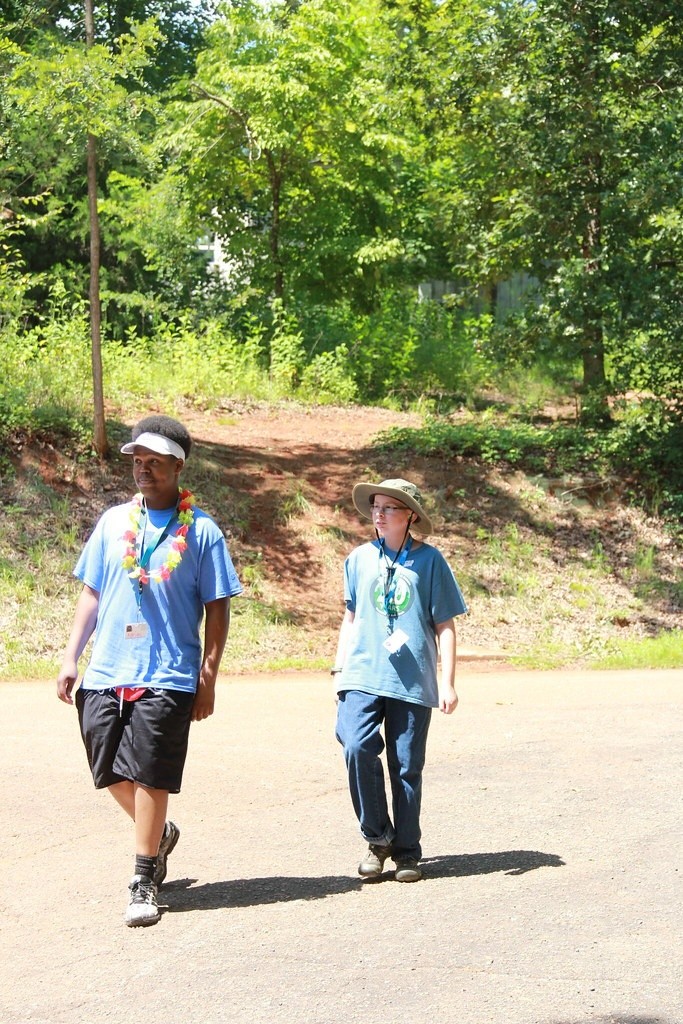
[331,667,343,675]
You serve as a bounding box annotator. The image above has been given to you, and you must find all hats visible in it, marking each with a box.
[352,477,434,536]
[119,432,185,467]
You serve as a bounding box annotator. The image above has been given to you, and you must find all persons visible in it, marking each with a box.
[57,416,243,928]
[331,478,467,884]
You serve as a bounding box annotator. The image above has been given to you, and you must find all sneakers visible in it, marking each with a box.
[125,873,160,928]
[394,858,421,881]
[151,819,180,886]
[358,841,393,877]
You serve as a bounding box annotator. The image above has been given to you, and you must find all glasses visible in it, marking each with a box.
[369,504,411,514]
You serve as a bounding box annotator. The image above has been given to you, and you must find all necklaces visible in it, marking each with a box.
[121,487,195,583]
